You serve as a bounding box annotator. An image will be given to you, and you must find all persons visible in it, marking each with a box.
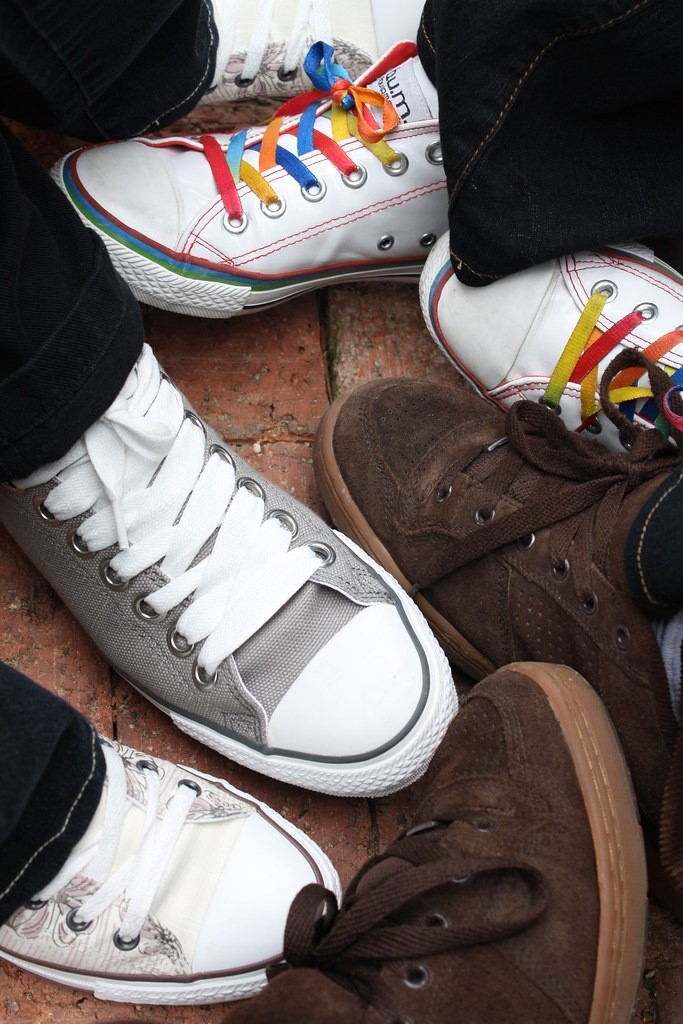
[47,0,682,452]
[0,1,457,1005]
[222,349,683,1024]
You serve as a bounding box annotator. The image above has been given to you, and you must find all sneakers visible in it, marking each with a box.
[314,376,682,835]
[48,43,451,318]
[199,2,374,106]
[418,228,682,471]
[221,662,646,1024]
[0,734,343,1007]
[3,344,458,797]
